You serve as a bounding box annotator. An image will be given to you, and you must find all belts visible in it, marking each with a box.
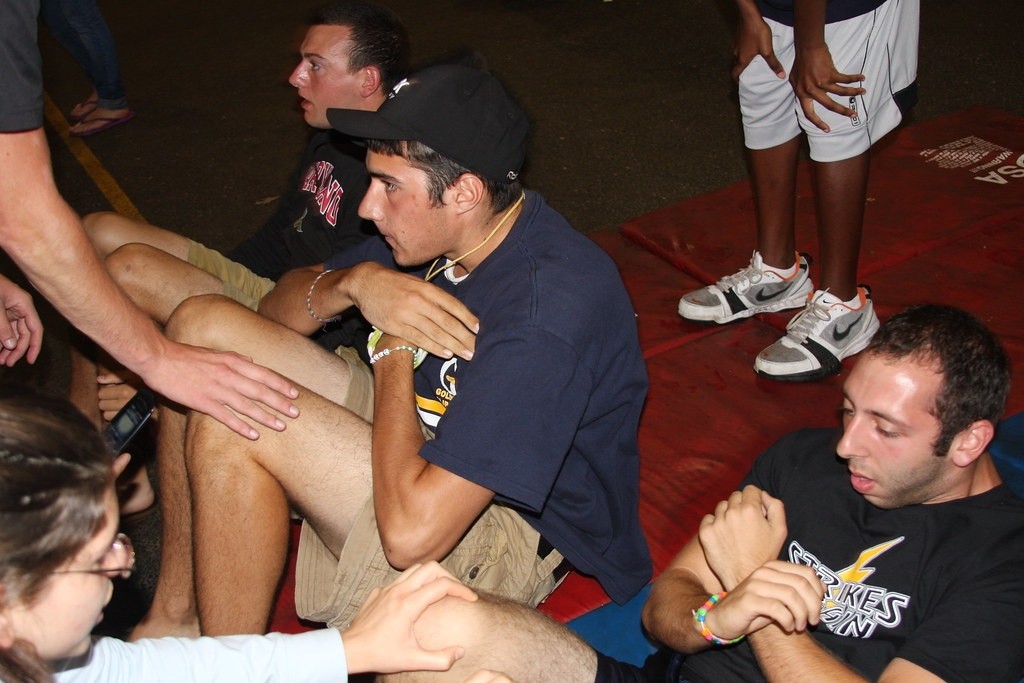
[537,537,571,583]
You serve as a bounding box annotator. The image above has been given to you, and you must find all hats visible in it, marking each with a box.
[325,61,530,186]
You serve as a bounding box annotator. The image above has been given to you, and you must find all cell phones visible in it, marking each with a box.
[100,387,155,459]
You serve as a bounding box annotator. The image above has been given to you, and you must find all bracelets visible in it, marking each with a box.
[369,346,417,365]
[306,269,341,322]
[691,591,744,645]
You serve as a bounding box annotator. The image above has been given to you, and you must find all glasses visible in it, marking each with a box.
[47,533,136,578]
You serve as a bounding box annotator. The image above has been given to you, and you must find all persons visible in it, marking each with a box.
[373,303,1024,683]
[0,0,649,683]
[678,1,921,383]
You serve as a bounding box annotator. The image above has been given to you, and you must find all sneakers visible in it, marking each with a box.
[754,283,880,382]
[678,249,814,325]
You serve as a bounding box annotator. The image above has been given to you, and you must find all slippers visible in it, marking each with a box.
[68,97,134,137]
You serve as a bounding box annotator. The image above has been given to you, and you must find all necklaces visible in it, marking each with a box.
[424,193,526,281]
